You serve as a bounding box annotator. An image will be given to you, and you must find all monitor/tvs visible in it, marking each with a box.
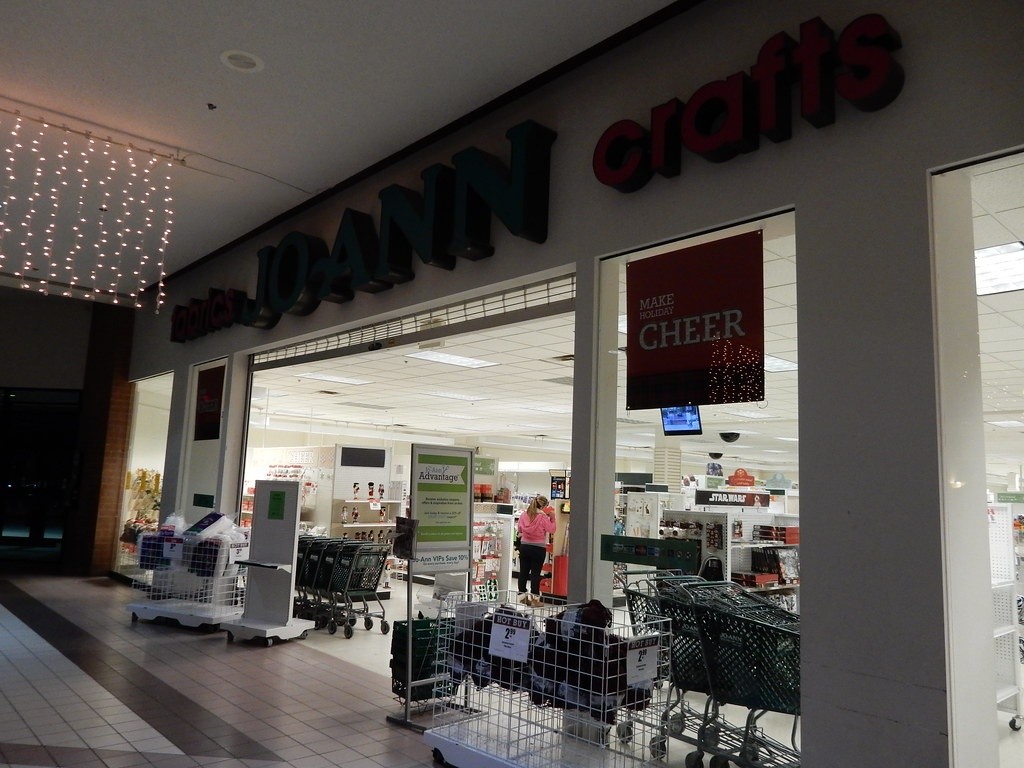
[660,405,703,436]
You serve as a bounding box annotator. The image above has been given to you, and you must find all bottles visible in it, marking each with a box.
[552,480,564,498]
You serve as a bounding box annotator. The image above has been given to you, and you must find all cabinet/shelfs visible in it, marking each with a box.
[986,491,1024,731]
[240,443,799,615]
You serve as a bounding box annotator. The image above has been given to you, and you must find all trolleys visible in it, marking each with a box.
[293,533,390,637]
[615,570,801,768]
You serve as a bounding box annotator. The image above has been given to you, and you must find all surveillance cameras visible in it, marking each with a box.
[719,433,740,443]
[709,453,723,460]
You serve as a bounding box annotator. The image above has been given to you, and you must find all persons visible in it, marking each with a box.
[343,529,393,545]
[379,506,386,522]
[383,560,391,588]
[340,506,361,525]
[353,482,385,501]
[518,495,557,608]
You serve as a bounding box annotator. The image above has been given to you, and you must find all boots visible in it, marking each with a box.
[518,592,532,606]
[530,594,544,608]
